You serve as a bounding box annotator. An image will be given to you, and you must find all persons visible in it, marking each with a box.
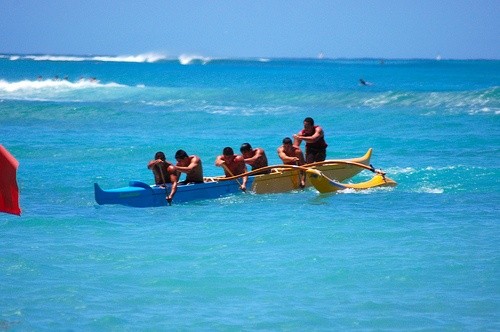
[239,142,268,174]
[292,117,328,164]
[148,151,178,202]
[216,146,248,190]
[174,149,203,183]
[278,137,306,188]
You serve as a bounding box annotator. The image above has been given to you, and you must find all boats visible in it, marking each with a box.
[94,148,373,208]
